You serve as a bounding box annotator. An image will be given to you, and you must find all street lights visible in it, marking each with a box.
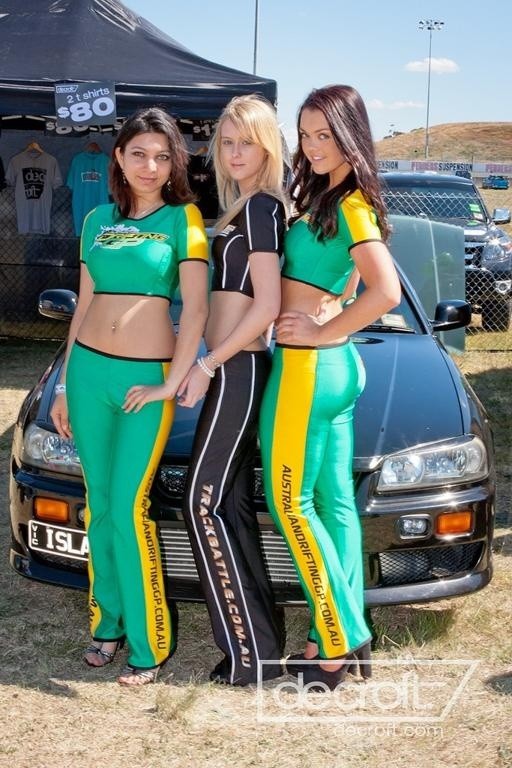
[417,18,445,161]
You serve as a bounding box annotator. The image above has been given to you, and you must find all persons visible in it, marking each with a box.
[260,85,401,690]
[50,107,209,685]
[177,94,288,688]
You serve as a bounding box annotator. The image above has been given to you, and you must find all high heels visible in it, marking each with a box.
[84,637,370,689]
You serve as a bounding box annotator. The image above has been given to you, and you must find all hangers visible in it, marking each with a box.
[86,142,101,153]
[25,142,44,155]
[194,146,209,156]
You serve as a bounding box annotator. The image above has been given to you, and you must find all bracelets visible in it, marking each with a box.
[54,384,65,395]
[207,351,224,368]
[197,357,215,378]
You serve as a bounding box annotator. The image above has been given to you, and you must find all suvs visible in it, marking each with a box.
[369,171,512,326]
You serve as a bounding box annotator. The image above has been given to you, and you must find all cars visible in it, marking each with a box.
[6,213,496,607]
[483,173,508,189]
[455,170,473,179]
